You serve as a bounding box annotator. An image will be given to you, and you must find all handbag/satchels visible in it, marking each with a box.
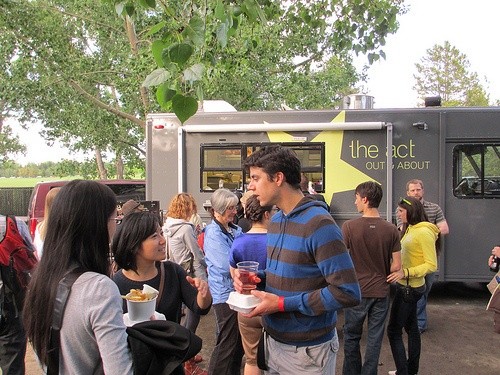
[180,258,195,278]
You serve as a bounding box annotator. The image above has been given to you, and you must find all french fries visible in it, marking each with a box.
[120,289,151,301]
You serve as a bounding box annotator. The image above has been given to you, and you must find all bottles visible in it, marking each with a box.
[490,245,500,273]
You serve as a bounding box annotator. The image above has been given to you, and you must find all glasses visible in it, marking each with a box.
[225,206,238,210]
[271,208,278,213]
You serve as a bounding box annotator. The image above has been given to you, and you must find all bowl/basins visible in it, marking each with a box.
[126,290,158,321]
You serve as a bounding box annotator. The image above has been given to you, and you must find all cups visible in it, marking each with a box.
[236,261,259,295]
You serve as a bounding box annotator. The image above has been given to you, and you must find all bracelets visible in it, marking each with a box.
[277,296,284,311]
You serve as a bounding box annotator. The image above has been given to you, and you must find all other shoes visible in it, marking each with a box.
[193,355,202,362]
[388,371,397,375]
[184,358,208,375]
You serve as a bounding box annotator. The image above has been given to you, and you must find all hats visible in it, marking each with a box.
[121,200,140,217]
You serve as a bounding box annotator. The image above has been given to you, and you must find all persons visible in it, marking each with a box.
[386,196,441,375]
[111,188,279,375]
[395,179,449,332]
[342,182,401,375]
[21,179,133,375]
[233,144,362,375]
[488,247,500,331]
[33,187,62,258]
[301,175,310,195]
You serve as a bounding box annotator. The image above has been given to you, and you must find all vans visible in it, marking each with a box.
[25,179,145,260]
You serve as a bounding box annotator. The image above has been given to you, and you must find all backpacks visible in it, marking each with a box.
[0,214,40,333]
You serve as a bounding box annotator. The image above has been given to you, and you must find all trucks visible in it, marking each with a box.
[145,96,500,288]
